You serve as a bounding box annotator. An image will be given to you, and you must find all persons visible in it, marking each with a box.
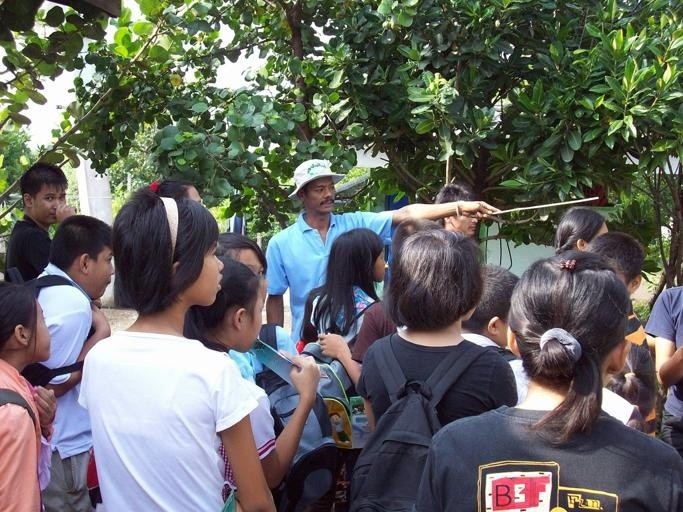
[0,160,683,512]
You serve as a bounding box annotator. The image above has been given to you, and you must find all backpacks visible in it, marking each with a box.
[256,286,375,512]
[349,333,488,512]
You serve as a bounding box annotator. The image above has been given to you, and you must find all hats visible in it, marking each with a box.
[288,159,345,202]
